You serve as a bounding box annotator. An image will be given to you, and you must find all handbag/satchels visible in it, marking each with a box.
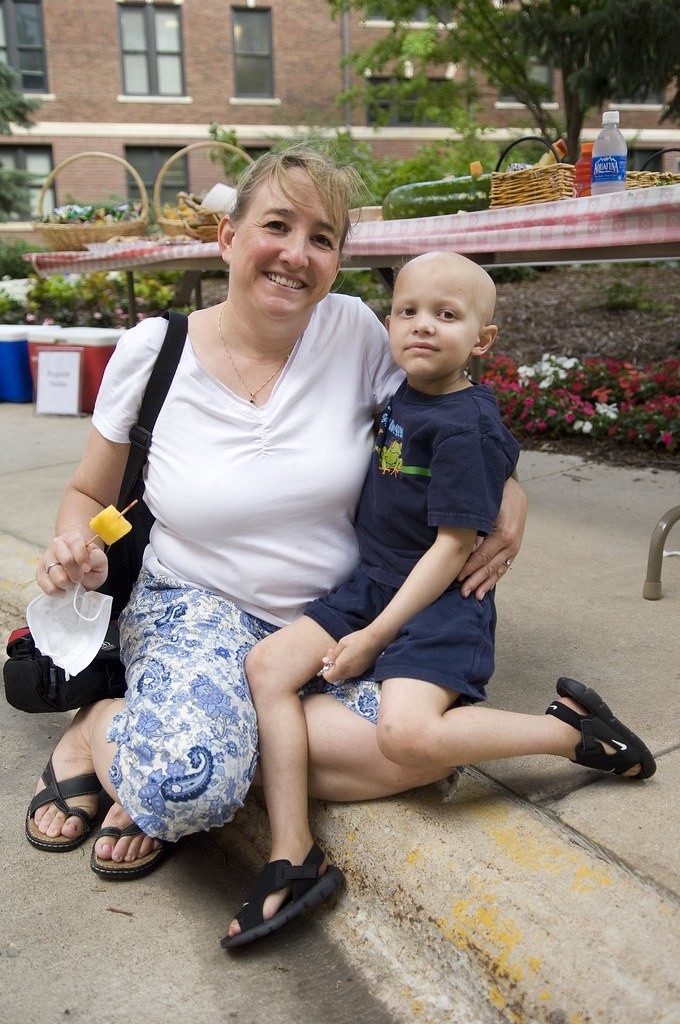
[3,622,128,716]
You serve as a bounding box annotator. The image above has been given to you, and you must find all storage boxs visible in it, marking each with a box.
[26,328,126,413]
[0,323,62,403]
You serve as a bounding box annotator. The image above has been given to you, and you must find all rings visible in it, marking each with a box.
[46,563,61,573]
[502,559,512,568]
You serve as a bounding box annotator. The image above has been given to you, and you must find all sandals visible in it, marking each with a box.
[25,751,102,854]
[220,841,343,953]
[544,675,656,781]
[90,794,165,877]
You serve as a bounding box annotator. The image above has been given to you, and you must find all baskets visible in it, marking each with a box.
[34,150,152,251]
[155,140,255,234]
[487,158,680,206]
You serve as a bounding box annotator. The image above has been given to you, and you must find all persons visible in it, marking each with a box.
[21,140,527,879]
[218,247,655,951]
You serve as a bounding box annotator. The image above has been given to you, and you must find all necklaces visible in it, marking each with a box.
[219,301,295,402]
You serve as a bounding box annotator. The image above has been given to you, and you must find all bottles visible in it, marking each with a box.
[591,110,628,196]
[573,143,594,198]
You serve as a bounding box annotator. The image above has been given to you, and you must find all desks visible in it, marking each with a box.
[22,181,680,601]
